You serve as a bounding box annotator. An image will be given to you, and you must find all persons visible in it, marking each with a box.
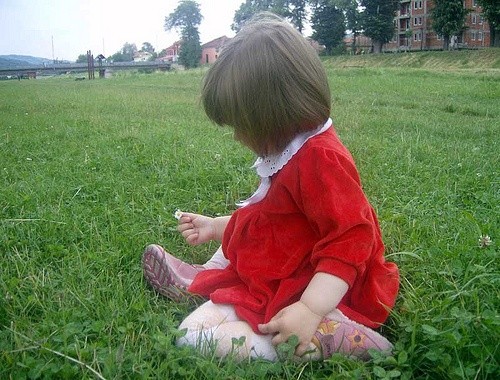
[355,46,362,55]
[141,11,399,367]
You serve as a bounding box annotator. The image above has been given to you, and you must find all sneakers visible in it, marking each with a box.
[311,308,396,363]
[141,244,207,303]
